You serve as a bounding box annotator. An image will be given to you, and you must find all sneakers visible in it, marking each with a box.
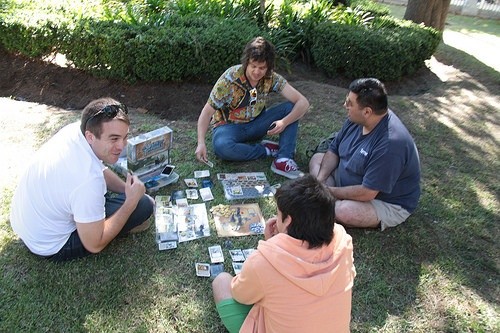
[269,158,304,180]
[262,140,280,157]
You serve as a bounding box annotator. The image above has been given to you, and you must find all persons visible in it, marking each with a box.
[308,78,421,231]
[210,174,357,333]
[9,96,155,263]
[194,36,306,179]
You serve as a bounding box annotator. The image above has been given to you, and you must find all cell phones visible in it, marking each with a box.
[210,263,225,281]
[268,123,276,130]
[160,165,176,178]
[202,179,213,188]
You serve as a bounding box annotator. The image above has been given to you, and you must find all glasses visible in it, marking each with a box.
[345,94,359,108]
[83,103,128,137]
[249,87,257,106]
